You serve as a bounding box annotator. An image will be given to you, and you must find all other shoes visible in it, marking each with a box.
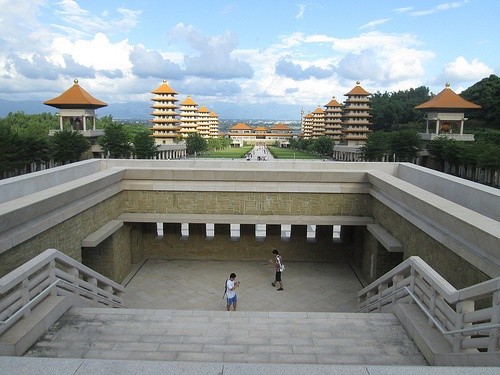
[276,288,283,291]
[271,283,276,287]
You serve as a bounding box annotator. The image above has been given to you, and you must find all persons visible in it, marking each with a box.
[270,249,284,290]
[244,146,269,161]
[226,272,240,311]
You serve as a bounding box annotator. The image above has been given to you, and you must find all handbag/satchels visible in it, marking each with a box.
[280,264,286,272]
